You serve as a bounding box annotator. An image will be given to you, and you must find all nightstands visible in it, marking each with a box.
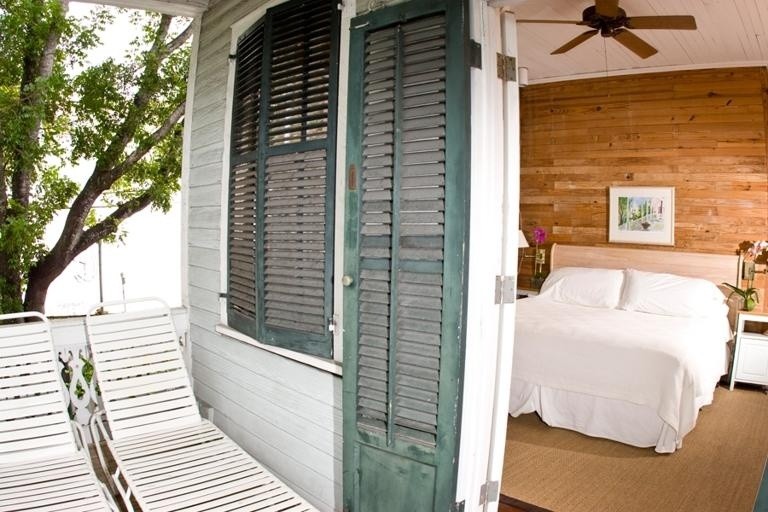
[729,312,768,391]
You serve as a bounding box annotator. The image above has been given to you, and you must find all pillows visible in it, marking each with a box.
[536,266,725,322]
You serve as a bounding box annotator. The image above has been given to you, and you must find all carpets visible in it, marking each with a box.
[501,382,767,512]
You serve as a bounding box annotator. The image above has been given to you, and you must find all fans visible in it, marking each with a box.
[514,0,697,59]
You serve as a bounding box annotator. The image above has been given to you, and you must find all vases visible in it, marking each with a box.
[531,277,544,289]
[744,297,755,311]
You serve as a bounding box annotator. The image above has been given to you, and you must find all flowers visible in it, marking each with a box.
[532,225,549,278]
[721,238,768,304]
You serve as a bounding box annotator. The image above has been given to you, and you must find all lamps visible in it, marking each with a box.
[518,229,530,249]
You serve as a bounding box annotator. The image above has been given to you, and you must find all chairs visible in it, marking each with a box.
[85,298,321,512]
[3,310,118,512]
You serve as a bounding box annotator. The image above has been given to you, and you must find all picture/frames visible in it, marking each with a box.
[608,185,676,247]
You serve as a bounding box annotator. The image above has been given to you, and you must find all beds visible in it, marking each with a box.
[509,243,744,455]
